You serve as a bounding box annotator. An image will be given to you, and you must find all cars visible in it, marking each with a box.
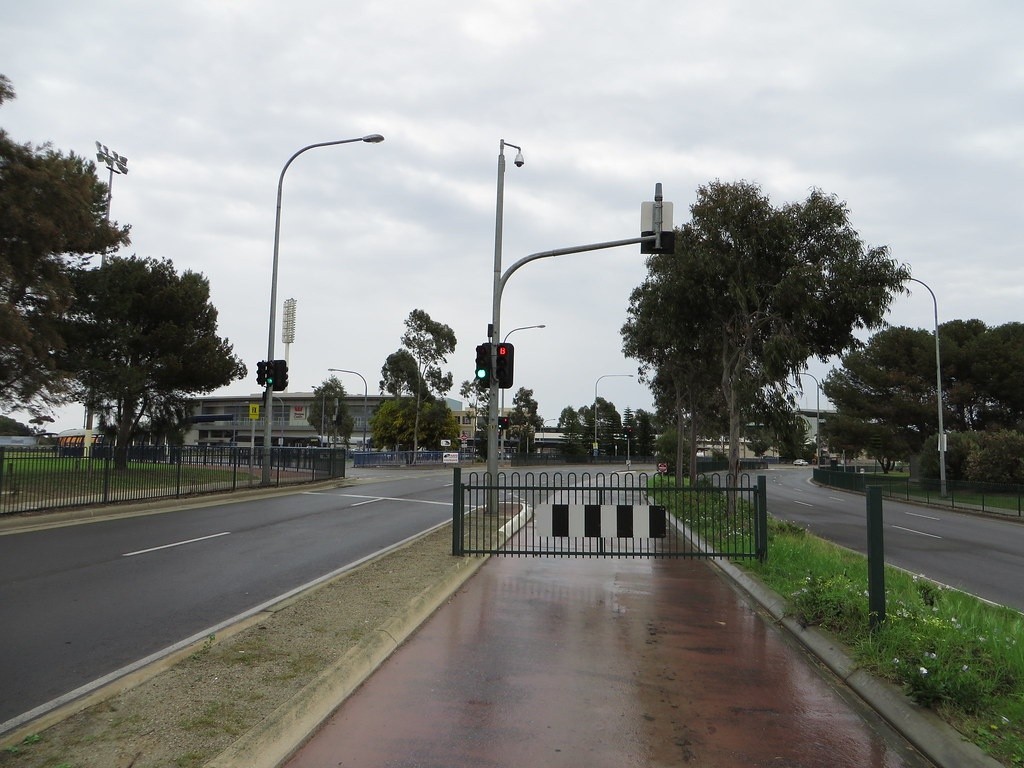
[793,459,808,467]
[348,448,363,452]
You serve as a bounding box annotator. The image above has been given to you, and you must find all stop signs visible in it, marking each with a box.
[658,463,667,473]
[461,434,467,440]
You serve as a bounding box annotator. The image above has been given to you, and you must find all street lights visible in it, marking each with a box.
[594,374,634,443]
[501,324,546,467]
[788,371,820,470]
[543,419,555,443]
[260,134,385,484]
[901,277,947,497]
[328,368,367,452]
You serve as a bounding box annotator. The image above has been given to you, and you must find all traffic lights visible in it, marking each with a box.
[267,361,275,386]
[496,343,512,381]
[275,360,288,391]
[256,361,266,386]
[502,417,509,430]
[627,427,632,438]
[474,343,491,388]
